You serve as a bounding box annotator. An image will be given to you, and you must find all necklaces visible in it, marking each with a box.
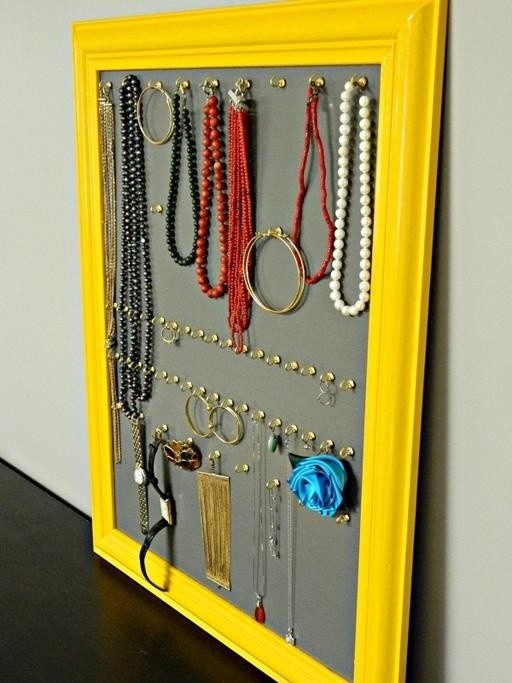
[330,80,374,318]
[290,83,336,287]
[116,75,156,422]
[250,416,267,624]
[225,87,255,354]
[195,83,230,300]
[98,81,124,468]
[165,84,200,267]
[284,435,297,647]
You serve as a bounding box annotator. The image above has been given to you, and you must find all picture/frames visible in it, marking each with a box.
[70,0,449,683]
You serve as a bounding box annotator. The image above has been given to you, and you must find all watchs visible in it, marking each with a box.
[130,415,150,537]
[140,430,176,593]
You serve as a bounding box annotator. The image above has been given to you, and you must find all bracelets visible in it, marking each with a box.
[135,81,175,145]
[209,404,245,446]
[195,455,234,592]
[265,485,282,561]
[185,393,214,440]
[242,228,307,318]
